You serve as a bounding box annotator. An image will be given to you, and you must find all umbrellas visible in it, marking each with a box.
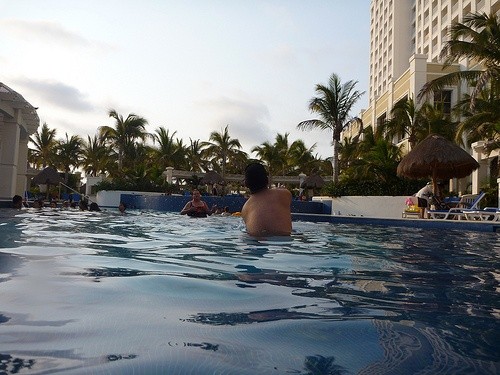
[397,132,479,197]
[301,173,325,196]
[203,171,225,184]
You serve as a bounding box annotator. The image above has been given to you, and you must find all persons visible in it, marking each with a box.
[13,187,230,219]
[241,163,293,237]
[416,182,434,218]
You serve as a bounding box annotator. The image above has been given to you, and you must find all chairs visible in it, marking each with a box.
[401,192,500,221]
